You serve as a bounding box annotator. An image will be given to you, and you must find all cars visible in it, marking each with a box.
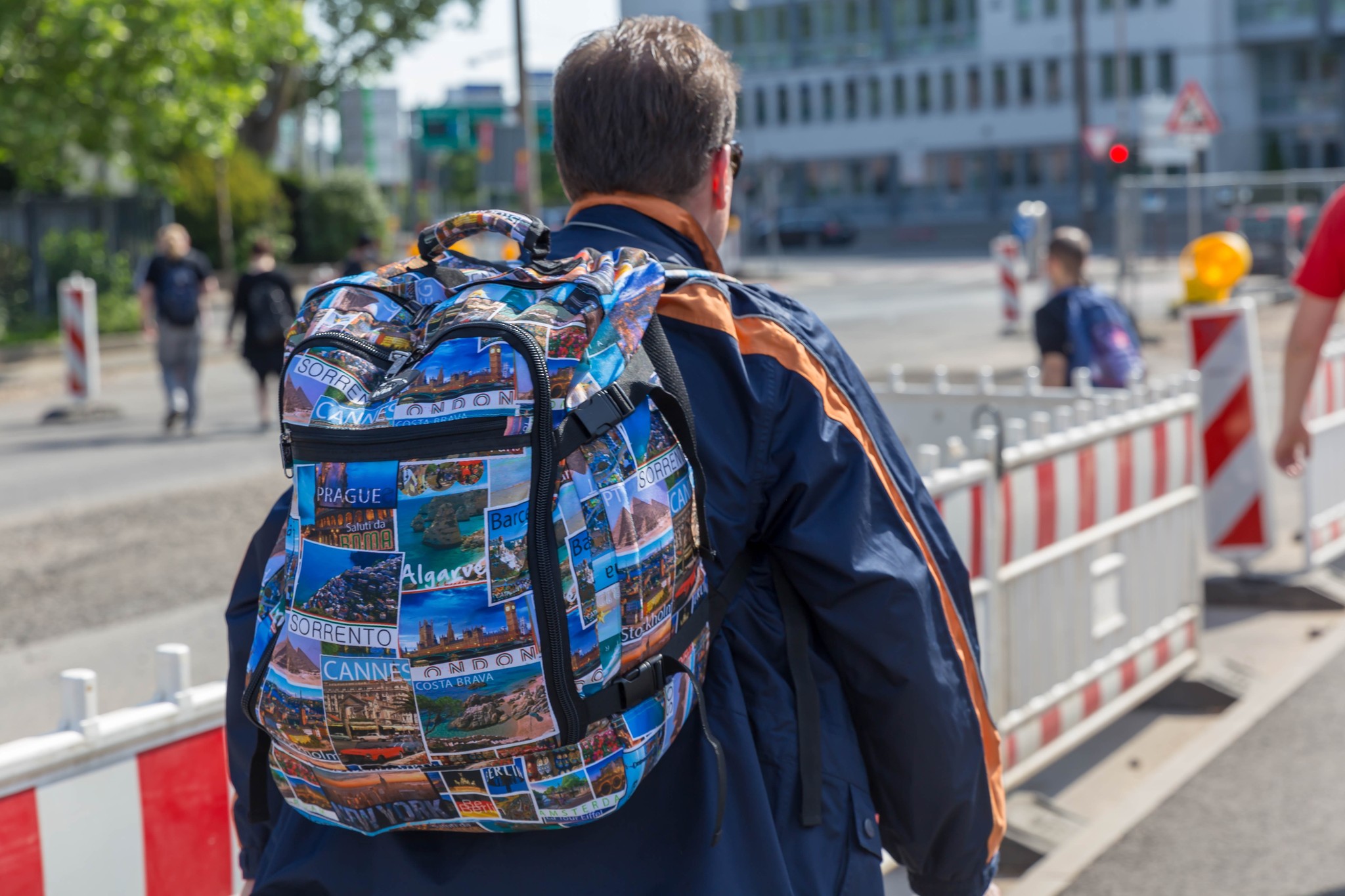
[1222,202,1319,276]
[746,205,857,252]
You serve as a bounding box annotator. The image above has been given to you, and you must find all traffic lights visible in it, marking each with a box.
[1105,135,1131,172]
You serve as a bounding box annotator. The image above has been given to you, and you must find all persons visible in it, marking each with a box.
[1272,189,1345,476]
[1030,225,1144,393]
[139,224,389,439]
[220,19,1007,896]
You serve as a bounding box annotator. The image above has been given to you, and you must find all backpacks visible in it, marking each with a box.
[156,266,204,326]
[245,278,295,349]
[1065,292,1148,393]
[242,209,732,846]
